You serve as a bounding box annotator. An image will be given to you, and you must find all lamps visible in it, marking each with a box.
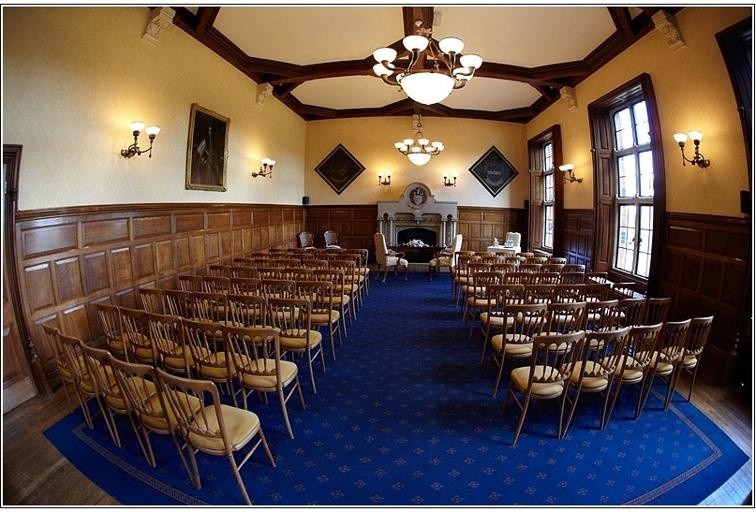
[252,158,276,178]
[377,171,392,186]
[372,7,483,106]
[443,172,458,188]
[673,130,710,169]
[120,121,161,159]
[393,113,445,166]
[558,164,583,184]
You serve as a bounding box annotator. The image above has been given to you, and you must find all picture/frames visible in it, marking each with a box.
[185,103,232,192]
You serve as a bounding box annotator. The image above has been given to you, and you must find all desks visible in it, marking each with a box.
[388,245,446,278]
[487,245,522,256]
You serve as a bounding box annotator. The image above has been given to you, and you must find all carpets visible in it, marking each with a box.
[41,271,750,506]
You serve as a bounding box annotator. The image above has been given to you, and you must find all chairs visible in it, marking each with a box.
[428,234,463,282]
[506,232,521,247]
[373,233,409,283]
